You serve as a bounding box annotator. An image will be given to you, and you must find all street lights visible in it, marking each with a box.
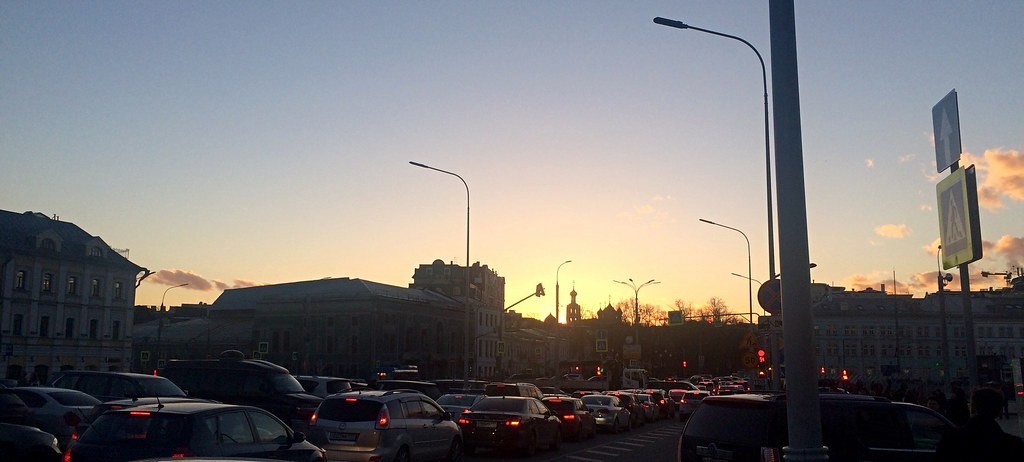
[407,160,470,389]
[613,279,663,344]
[653,17,783,391]
[731,272,766,316]
[157,283,188,343]
[700,219,755,394]
[555,259,571,377]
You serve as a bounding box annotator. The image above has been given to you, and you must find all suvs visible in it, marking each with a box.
[677,386,958,462]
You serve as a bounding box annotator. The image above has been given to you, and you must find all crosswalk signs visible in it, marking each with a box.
[936,165,973,270]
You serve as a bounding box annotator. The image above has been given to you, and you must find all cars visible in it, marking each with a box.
[0,350,756,462]
[541,395,597,443]
[458,394,564,457]
[581,394,632,435]
[308,386,463,462]
[63,401,329,462]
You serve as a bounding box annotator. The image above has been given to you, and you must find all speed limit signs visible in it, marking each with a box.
[741,352,759,369]
